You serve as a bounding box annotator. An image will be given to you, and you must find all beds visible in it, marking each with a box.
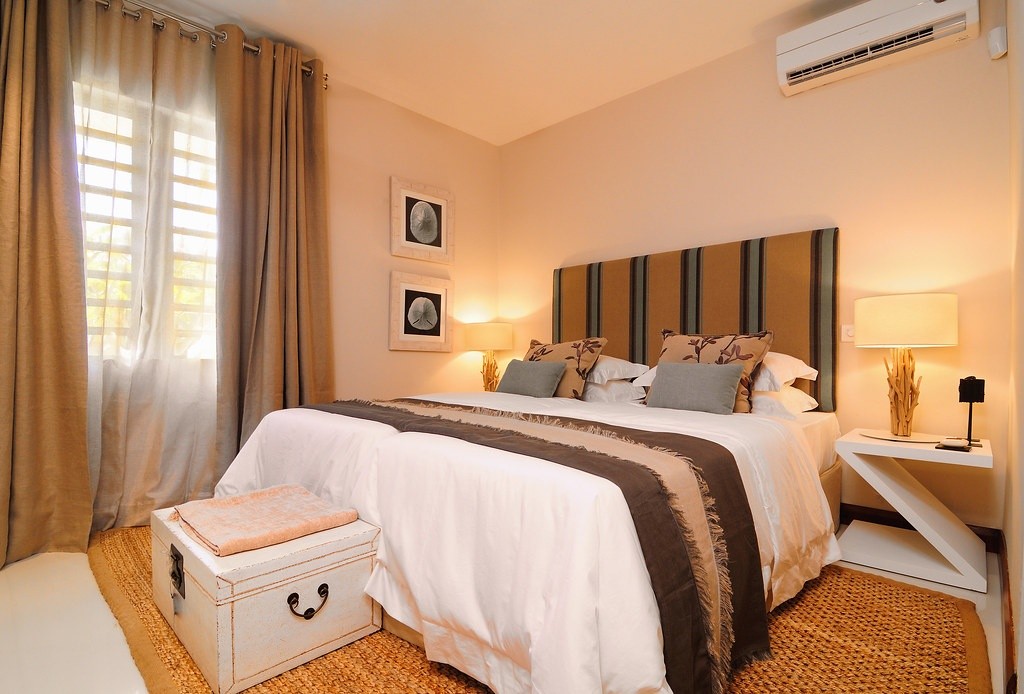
[210,227,844,694]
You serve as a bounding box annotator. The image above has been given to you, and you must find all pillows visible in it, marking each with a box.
[747,384,821,421]
[495,358,568,399]
[580,379,647,406]
[586,354,649,386]
[523,336,609,401]
[644,362,745,414]
[632,350,820,394]
[640,328,774,416]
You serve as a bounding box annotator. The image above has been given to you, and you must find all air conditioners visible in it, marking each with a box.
[774,0,980,96]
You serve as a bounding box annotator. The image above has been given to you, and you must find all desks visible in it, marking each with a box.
[828,428,994,594]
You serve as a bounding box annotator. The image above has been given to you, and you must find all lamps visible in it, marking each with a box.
[854,292,958,442]
[461,321,514,393]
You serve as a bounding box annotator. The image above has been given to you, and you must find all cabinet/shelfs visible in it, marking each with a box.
[149,502,385,694]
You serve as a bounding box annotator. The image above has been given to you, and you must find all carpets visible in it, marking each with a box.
[87,520,994,694]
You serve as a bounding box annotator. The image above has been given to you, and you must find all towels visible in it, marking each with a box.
[166,482,358,557]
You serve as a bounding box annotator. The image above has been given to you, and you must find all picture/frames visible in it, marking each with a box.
[390,175,453,266]
[388,269,454,354]
